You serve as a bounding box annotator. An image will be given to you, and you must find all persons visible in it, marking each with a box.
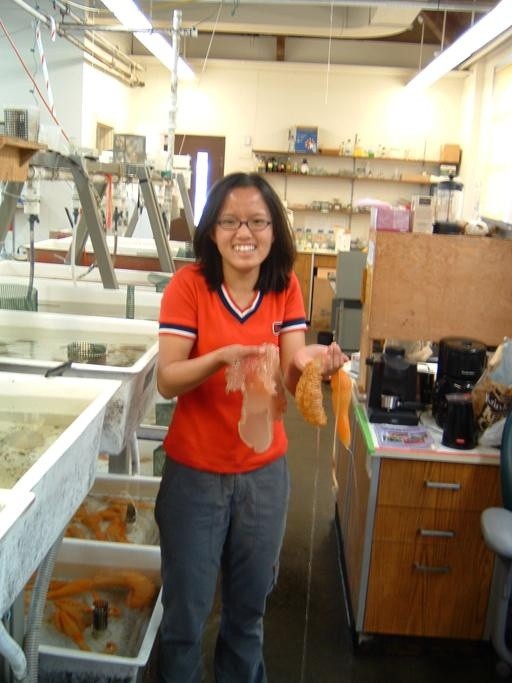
[156,172,349,683]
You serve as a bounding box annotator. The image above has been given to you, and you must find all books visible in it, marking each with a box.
[375,423,436,452]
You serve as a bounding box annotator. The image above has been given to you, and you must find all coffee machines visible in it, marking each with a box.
[366,345,423,426]
[436,335,489,431]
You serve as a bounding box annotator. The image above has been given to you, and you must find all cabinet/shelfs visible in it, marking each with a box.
[329,399,510,658]
[250,146,460,252]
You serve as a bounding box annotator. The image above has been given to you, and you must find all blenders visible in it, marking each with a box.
[429,174,466,234]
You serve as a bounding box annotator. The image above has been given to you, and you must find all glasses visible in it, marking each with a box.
[215,216,273,230]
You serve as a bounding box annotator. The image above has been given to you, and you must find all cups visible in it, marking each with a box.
[442,397,479,451]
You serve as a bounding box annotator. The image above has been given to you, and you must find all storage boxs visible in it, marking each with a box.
[309,265,339,331]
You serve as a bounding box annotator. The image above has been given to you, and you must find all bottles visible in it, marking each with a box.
[336,138,386,159]
[312,199,342,214]
[293,228,335,253]
[255,153,310,176]
